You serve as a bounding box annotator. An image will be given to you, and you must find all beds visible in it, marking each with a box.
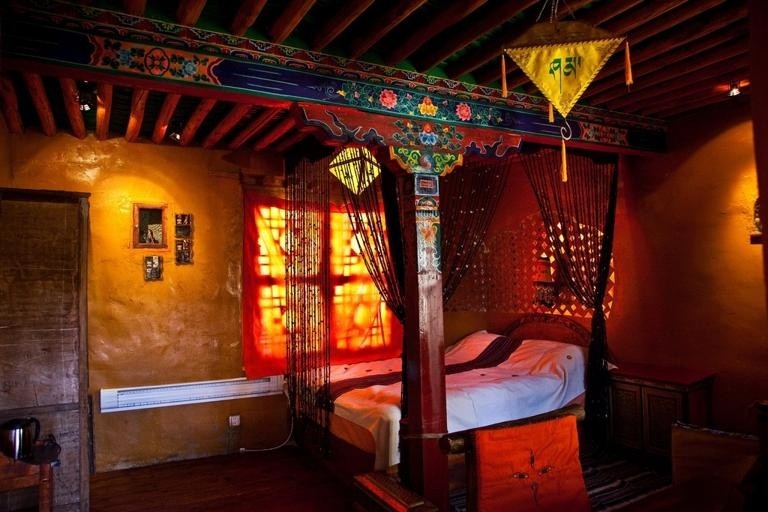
[286,313,615,484]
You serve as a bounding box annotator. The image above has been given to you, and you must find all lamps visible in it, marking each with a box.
[167,129,180,142]
[728,79,740,97]
[748,197,763,245]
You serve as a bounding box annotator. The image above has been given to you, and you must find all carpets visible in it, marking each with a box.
[354,444,673,512]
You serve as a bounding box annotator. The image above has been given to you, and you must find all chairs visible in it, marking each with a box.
[470,413,593,512]
[671,420,762,512]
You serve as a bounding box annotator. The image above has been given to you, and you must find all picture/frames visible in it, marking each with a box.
[133,202,167,249]
[175,239,194,265]
[174,213,192,237]
[143,254,164,280]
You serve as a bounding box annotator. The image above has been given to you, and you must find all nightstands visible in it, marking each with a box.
[609,355,718,460]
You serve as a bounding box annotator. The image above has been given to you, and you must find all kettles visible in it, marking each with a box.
[0,416,41,465]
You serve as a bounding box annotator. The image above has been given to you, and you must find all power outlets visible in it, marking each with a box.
[229,414,241,427]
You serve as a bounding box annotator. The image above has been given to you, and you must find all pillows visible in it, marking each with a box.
[501,339,591,380]
[445,328,503,360]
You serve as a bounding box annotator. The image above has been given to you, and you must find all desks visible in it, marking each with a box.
[0,439,50,511]
